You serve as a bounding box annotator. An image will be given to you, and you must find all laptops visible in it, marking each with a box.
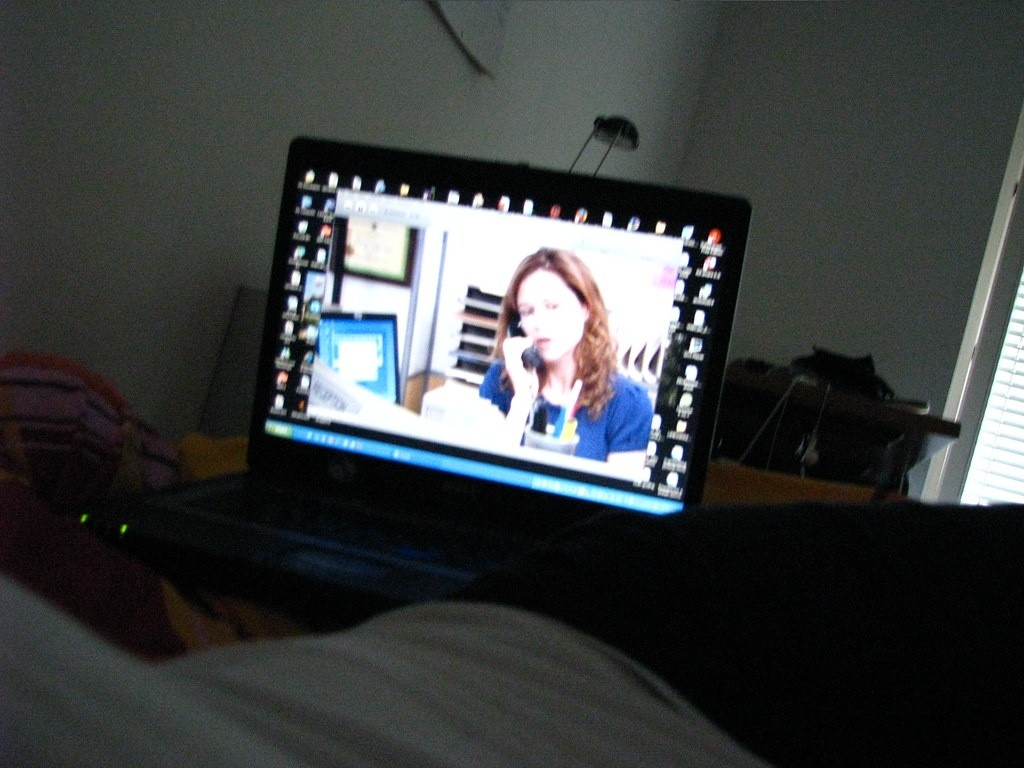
[69,135,754,637]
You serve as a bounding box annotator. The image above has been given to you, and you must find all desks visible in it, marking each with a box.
[75,452,895,622]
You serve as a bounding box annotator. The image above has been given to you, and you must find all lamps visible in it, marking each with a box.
[570,114,640,177]
